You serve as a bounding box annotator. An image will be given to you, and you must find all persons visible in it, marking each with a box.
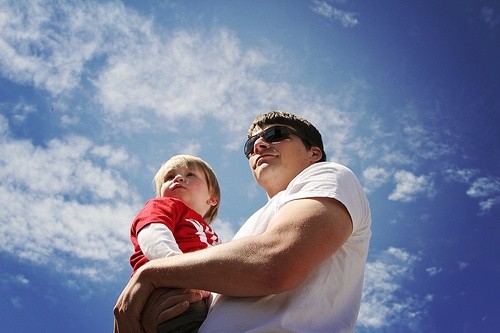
[130,154,221,333]
[113,111,372,333]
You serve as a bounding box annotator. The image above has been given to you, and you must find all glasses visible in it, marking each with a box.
[244,125,312,160]
